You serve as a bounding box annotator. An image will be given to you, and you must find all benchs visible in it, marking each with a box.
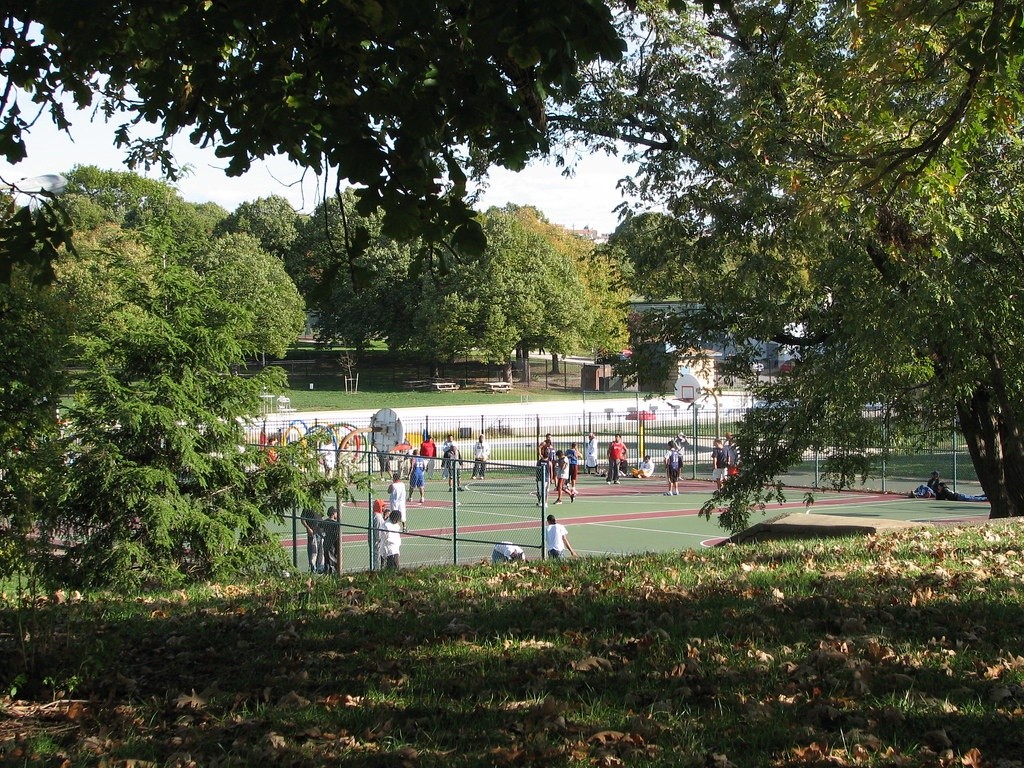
[438,387,457,393]
[415,385,430,390]
[400,384,419,388]
[491,388,514,391]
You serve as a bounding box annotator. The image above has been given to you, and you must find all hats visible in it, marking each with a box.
[327,506,338,516]
[677,432,684,439]
[373,499,385,512]
[427,435,431,439]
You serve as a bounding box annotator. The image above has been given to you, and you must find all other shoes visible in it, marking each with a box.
[408,498,412,501]
[403,527,409,533]
[449,487,453,492]
[614,480,620,484]
[607,482,611,485]
[678,477,684,481]
[571,489,578,494]
[537,503,549,507]
[471,476,477,479]
[672,489,680,495]
[663,491,673,496]
[481,476,484,479]
[553,498,562,504]
[554,488,558,491]
[457,486,464,491]
[420,498,424,503]
[570,494,575,502]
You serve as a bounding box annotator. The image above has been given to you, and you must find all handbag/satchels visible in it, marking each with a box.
[716,447,728,469]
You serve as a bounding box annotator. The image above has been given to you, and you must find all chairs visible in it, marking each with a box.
[275,395,297,413]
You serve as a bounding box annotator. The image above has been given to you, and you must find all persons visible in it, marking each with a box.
[605,433,628,485]
[387,473,409,533]
[663,432,690,496]
[632,455,655,479]
[535,434,583,507]
[586,433,599,474]
[368,499,402,571]
[936,483,989,502]
[492,541,526,564]
[712,432,739,495]
[471,435,490,480]
[442,435,465,492]
[299,499,340,574]
[379,435,436,503]
[266,439,280,465]
[908,471,939,498]
[545,514,577,559]
[318,437,352,485]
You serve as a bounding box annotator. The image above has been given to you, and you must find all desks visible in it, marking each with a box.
[484,382,511,394]
[403,380,428,392]
[431,383,456,391]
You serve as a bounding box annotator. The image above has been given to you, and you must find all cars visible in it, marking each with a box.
[748,360,764,376]
[780,360,796,375]
[625,410,656,421]
